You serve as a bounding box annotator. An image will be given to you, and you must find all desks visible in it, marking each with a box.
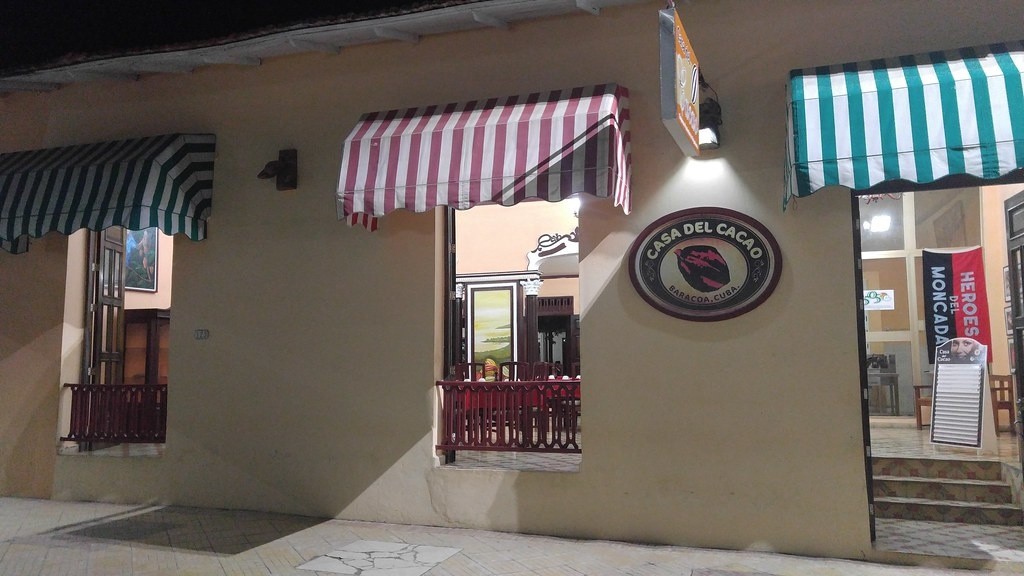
[523,381,582,434]
[868,373,899,415]
[455,382,523,434]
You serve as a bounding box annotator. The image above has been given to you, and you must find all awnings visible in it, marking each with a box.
[334,82,632,233]
[0,134,216,254]
[782,40,1024,214]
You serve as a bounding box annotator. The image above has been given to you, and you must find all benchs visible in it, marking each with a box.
[911,374,1014,439]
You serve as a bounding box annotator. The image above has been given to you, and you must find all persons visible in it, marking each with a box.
[950,340,976,357]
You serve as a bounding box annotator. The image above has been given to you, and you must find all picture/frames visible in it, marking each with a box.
[105,227,158,293]
[1002,263,1022,302]
[471,286,514,381]
[1008,338,1015,370]
[1004,306,1013,335]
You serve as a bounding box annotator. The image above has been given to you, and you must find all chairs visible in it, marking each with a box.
[454,362,581,434]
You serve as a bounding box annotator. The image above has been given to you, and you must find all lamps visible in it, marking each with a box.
[699,96,720,149]
[564,197,580,218]
[855,193,904,204]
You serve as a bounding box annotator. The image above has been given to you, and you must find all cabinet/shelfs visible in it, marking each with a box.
[121,307,170,403]
